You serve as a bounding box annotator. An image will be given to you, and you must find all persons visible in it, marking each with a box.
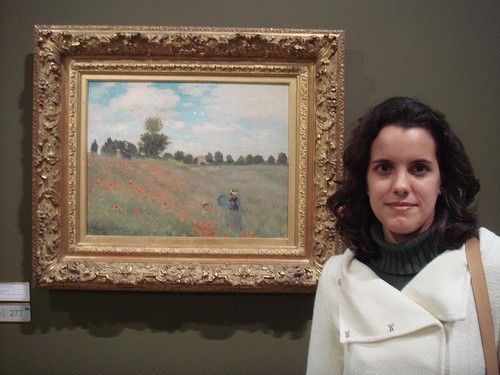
[307,97,500,375]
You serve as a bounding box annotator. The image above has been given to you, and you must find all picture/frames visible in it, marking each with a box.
[30,23,346,296]
